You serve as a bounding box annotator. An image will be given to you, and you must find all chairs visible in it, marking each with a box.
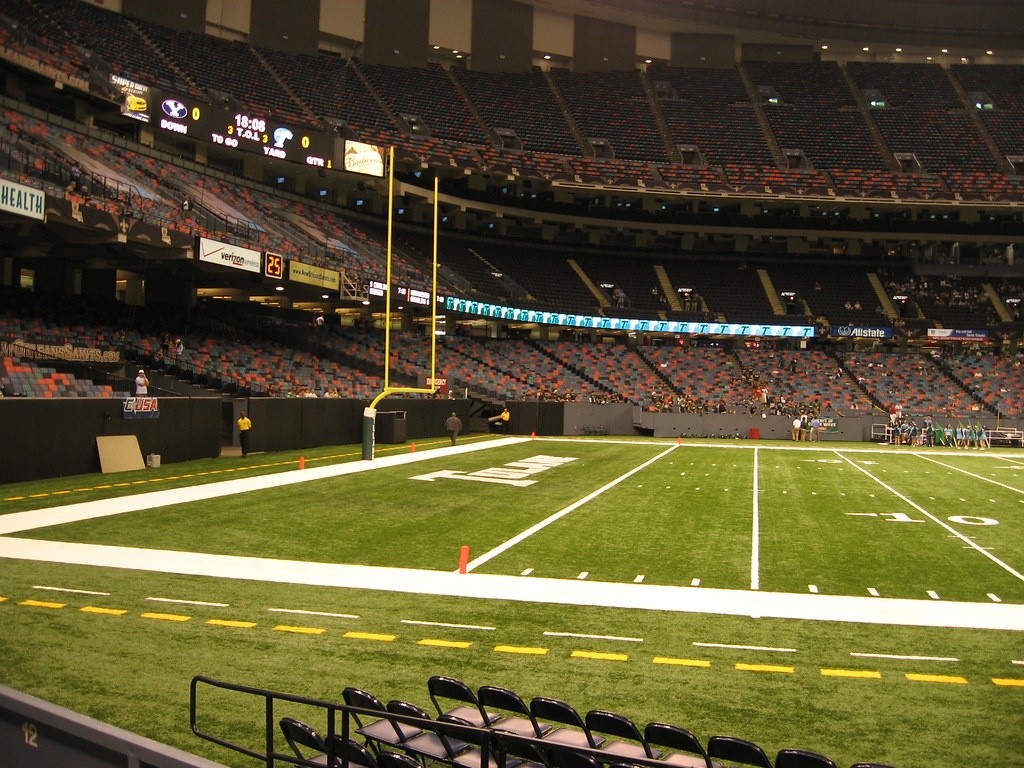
[280,675,836,768]
[0,0,1024,414]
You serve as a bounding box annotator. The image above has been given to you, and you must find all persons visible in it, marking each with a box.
[501,409,510,435]
[979,425,986,450]
[944,423,953,448]
[69,119,1024,427]
[792,417,801,442]
[236,411,251,458]
[956,424,978,450]
[444,412,462,446]
[135,369,149,397]
[801,416,820,443]
[892,415,935,448]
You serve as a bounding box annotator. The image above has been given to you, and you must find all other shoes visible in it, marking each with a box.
[957,446,985,451]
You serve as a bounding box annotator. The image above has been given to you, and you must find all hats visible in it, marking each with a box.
[176,339,180,342]
[139,370,144,373]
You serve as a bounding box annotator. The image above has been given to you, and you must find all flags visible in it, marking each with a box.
[935,419,983,447]
